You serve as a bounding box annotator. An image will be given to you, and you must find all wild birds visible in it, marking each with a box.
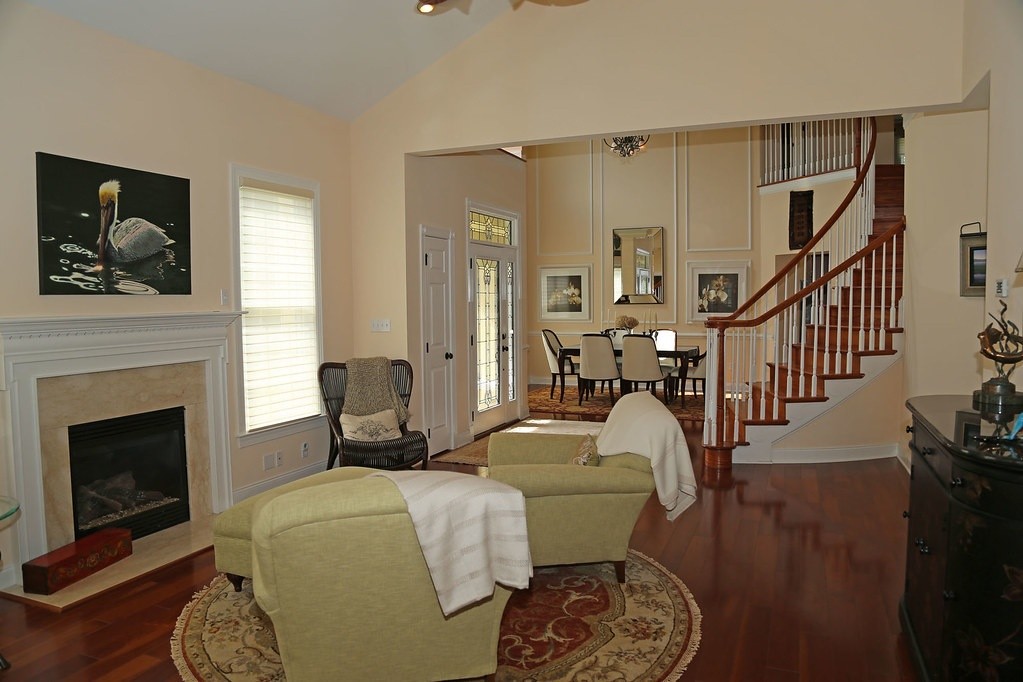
[95,179,176,265]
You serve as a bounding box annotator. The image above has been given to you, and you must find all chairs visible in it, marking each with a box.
[318,358,428,472]
[542,328,706,408]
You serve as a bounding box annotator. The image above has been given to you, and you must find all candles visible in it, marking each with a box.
[644,312,646,331]
[655,312,657,332]
[615,311,616,331]
[608,309,609,328]
[649,308,652,328]
[601,310,602,330]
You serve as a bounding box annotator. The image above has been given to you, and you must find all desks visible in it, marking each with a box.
[557,346,699,410]
[0,493,21,671]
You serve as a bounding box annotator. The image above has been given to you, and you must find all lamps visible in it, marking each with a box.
[602,134,650,157]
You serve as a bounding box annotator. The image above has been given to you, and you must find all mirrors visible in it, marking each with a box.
[613,226,664,304]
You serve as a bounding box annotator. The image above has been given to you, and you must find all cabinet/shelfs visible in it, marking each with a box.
[899,409,1023,682]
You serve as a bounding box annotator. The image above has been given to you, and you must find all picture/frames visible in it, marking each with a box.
[536,268,590,321]
[959,233,988,297]
[686,258,752,324]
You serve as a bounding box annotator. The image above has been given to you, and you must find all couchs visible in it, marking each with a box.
[214,464,533,682]
[488,391,698,584]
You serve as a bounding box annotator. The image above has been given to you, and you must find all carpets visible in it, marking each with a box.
[528,383,705,420]
[432,418,607,467]
[169,544,703,682]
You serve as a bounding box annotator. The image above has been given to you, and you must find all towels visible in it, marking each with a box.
[595,390,698,523]
[363,469,534,618]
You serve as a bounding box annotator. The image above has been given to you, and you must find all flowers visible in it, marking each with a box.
[615,315,639,334]
